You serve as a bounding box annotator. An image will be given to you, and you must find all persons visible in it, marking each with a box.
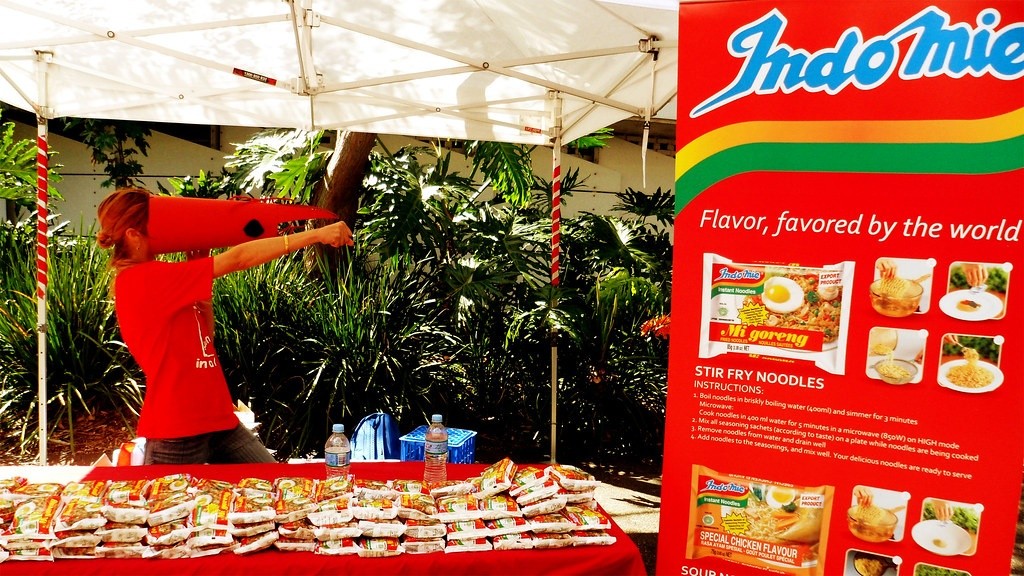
[97,190,355,466]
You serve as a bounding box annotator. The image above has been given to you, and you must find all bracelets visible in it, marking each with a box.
[284,235,289,256]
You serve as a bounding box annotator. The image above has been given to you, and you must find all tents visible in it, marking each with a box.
[0,1,679,465]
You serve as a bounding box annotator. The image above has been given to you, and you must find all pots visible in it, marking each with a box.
[853,552,896,576]
[870,271,932,317]
[847,503,905,543]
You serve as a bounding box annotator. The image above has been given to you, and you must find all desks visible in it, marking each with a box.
[0,462,645,576]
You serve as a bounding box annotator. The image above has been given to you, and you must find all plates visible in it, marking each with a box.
[938,290,1003,321]
[911,519,972,556]
[938,358,1004,393]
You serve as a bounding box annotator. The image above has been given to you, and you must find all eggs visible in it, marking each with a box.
[766,487,795,509]
[761,276,803,314]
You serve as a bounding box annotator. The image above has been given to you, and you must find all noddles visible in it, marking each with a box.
[726,499,823,562]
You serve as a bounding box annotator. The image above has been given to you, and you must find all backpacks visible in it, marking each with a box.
[350,411,400,462]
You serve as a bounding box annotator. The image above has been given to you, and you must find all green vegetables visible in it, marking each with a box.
[945,337,1000,361]
[916,564,968,576]
[924,501,979,530]
[950,266,1008,291]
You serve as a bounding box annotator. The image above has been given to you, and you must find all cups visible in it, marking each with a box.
[766,486,796,508]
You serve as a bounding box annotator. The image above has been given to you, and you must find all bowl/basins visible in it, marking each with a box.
[873,359,918,385]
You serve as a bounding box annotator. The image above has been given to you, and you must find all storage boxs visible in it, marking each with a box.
[399,426,477,463]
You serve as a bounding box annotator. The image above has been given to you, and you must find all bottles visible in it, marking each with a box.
[324,424,352,478]
[422,414,448,488]
